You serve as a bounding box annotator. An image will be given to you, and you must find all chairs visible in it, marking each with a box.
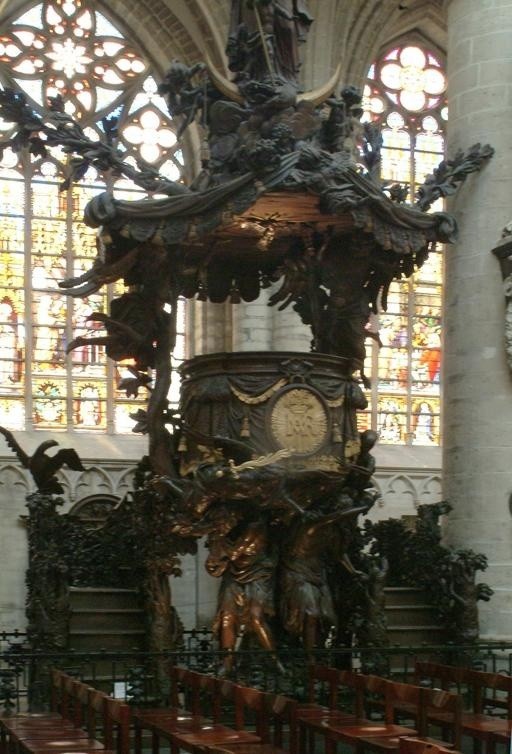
[2,660,511,754]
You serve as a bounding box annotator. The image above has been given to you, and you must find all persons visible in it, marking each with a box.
[225,0,314,95]
